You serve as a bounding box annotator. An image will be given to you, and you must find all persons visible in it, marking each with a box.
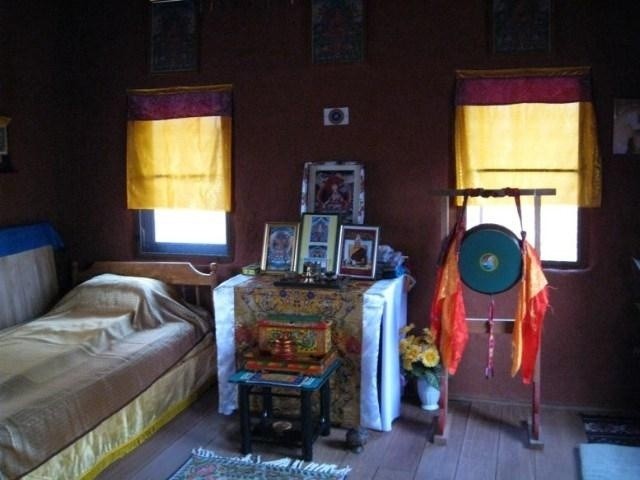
[350,233,366,265]
[317,173,352,209]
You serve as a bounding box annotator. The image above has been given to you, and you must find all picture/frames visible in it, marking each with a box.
[260,157,382,282]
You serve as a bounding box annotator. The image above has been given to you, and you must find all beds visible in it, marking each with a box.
[1,257,217,479]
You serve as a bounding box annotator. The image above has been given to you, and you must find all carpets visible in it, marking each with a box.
[163,447,354,479]
[578,410,640,445]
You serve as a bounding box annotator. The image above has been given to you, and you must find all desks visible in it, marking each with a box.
[213,269,407,463]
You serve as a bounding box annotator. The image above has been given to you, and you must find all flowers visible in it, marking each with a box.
[397,320,442,391]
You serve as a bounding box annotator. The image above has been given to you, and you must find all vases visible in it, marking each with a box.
[415,375,441,410]
